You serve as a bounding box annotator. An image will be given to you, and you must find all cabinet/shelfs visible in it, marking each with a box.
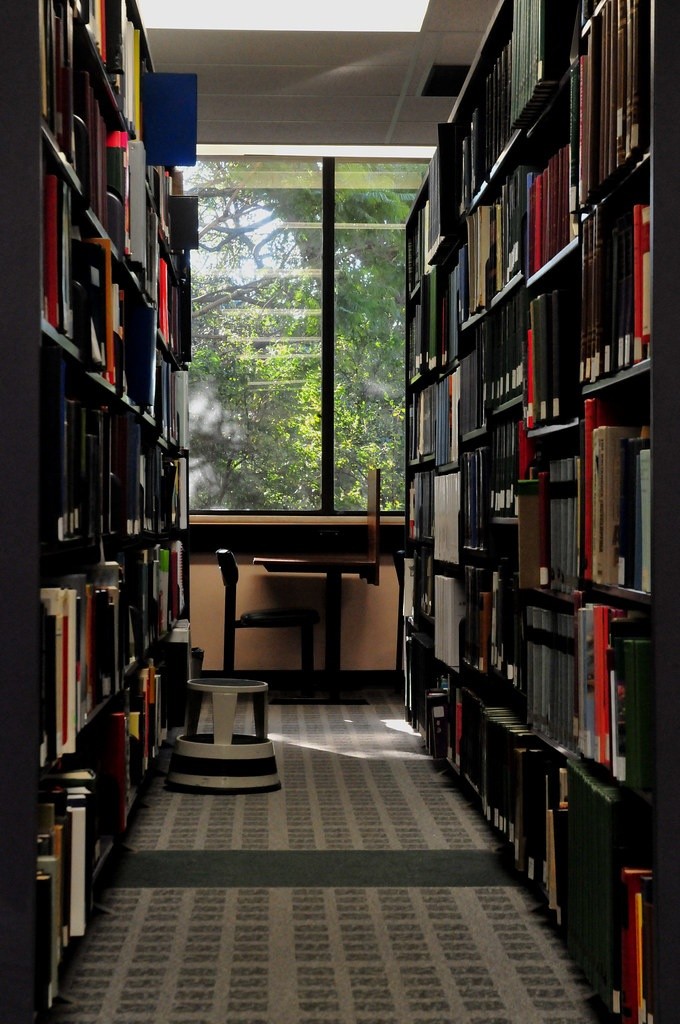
[405,0,680,1024]
[0,0,192,1024]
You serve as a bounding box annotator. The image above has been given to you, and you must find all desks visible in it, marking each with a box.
[251,468,381,706]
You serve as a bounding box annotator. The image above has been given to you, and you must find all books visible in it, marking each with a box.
[38,0,187,1009]
[399,0,659,1023]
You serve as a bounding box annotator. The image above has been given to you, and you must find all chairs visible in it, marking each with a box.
[215,549,322,697]
